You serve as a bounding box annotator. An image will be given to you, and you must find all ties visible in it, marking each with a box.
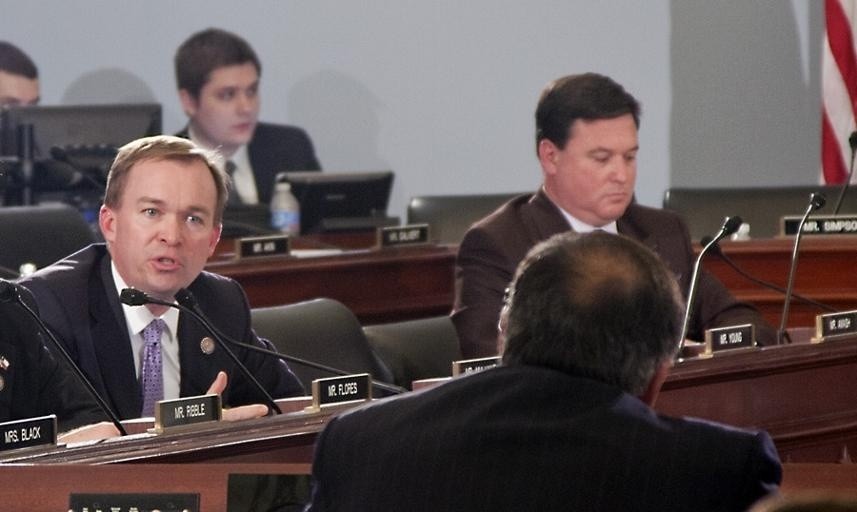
[138,318,166,418]
[223,160,245,208]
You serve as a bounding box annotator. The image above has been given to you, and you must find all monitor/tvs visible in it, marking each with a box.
[4,103,163,215]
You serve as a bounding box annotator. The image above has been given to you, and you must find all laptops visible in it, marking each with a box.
[274,170,391,230]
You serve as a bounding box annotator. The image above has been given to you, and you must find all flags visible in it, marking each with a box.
[820,0,857,189]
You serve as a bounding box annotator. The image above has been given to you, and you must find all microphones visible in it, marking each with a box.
[774,193,826,343]
[118,286,281,416]
[833,131,857,215]
[669,215,740,362]
[699,234,839,313]
[50,144,107,195]
[174,288,404,396]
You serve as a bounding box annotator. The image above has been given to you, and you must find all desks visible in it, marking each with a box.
[196,219,855,347]
[0,308,855,511]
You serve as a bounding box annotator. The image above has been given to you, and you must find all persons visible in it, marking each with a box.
[17,135,301,422]
[173,28,322,236]
[449,74,776,362]
[0,43,42,109]
[305,232,783,512]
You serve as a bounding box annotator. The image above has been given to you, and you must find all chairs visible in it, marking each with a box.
[406,188,534,243]
[356,309,463,382]
[663,183,855,242]
[242,299,377,392]
[1,203,95,432]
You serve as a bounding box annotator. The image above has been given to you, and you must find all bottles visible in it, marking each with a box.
[733,223,752,241]
[270,182,300,246]
